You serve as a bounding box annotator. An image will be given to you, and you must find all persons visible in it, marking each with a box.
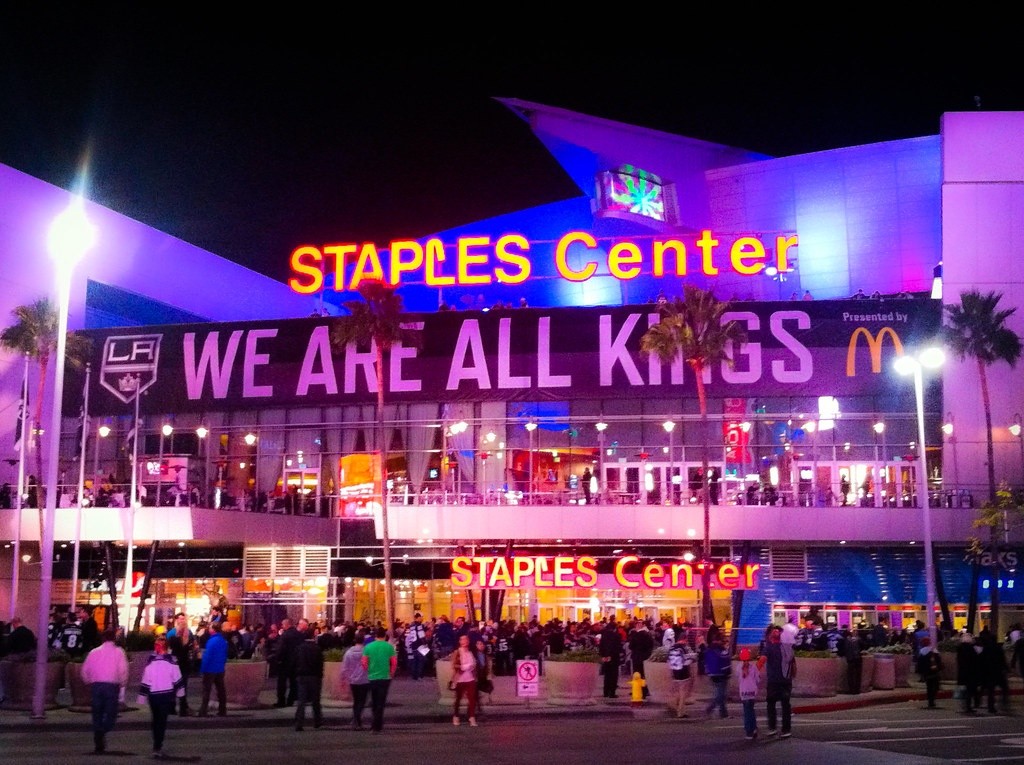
[0,475,335,517]
[581,468,591,505]
[694,466,871,506]
[0,606,1024,758]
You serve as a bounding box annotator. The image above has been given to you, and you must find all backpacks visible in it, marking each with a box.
[667,645,686,670]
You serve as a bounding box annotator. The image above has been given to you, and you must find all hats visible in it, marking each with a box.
[740,648,751,660]
[920,637,932,645]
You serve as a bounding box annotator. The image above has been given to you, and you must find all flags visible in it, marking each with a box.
[13,356,32,452]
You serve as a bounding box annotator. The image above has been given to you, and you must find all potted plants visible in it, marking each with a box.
[65,651,133,712]
[0,647,70,711]
[792,641,917,698]
[644,646,696,704]
[319,649,372,708]
[208,658,269,709]
[544,645,604,706]
[938,638,960,679]
[434,657,491,706]
[729,647,767,701]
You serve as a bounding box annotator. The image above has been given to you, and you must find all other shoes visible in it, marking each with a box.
[780,732,792,737]
[178,707,196,717]
[769,730,778,734]
[468,717,478,728]
[453,716,460,725]
[151,750,163,757]
